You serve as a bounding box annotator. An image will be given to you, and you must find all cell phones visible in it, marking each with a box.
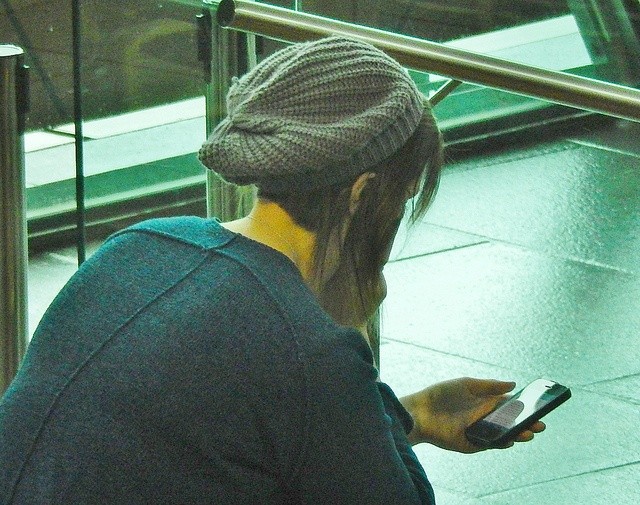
[464,378,571,447]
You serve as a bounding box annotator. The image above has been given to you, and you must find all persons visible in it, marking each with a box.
[1,37,547,504]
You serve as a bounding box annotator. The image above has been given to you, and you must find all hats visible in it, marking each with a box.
[197,34,425,198]
